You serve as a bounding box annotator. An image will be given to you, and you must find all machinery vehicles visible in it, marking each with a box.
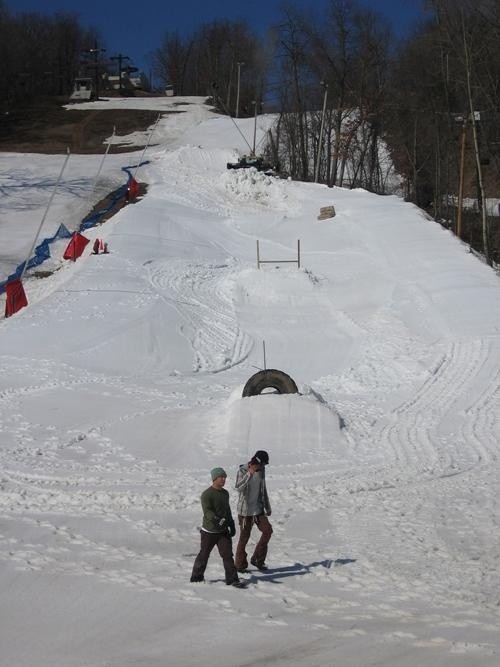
[69,77,96,103]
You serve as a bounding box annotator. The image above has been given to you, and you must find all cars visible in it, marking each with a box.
[227,155,272,170]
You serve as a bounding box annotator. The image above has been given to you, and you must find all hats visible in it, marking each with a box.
[210,468,227,481]
[252,450,270,464]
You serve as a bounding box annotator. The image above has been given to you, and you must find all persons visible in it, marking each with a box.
[188,464,246,588]
[233,450,273,572]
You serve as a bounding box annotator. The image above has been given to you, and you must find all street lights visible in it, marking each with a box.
[251,100,264,156]
[313,79,329,183]
[236,61,245,118]
[89,48,106,100]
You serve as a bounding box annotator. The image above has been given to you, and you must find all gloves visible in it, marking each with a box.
[219,517,236,537]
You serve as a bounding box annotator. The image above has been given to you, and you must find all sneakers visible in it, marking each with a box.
[230,579,245,589]
[250,556,268,571]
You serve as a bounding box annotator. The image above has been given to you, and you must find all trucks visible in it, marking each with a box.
[164,84,174,97]
[131,80,143,90]
